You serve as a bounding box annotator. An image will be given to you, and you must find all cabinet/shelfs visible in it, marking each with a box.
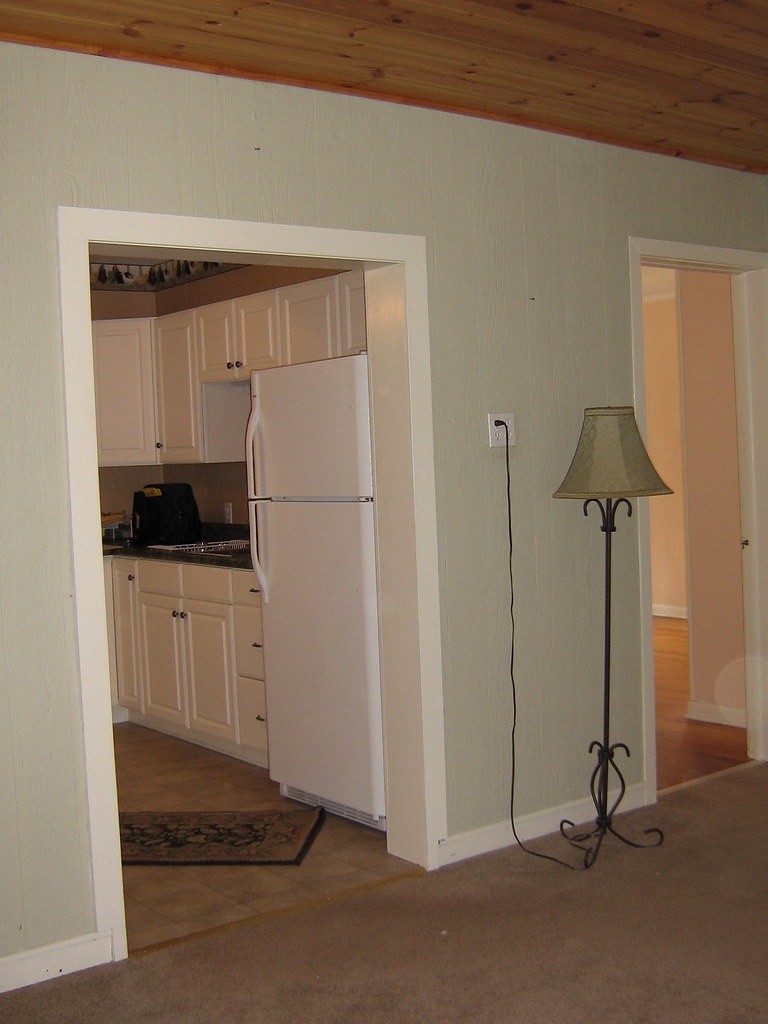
[102,522,269,768]
[89,270,366,464]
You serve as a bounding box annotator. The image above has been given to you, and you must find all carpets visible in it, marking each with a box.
[119,805,326,865]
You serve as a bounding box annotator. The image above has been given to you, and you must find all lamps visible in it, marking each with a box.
[552,406,677,868]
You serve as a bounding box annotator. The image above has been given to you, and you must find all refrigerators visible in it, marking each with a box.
[245,356,387,832]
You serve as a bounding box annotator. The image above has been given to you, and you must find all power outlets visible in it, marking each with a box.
[487,411,516,448]
[224,502,232,524]
